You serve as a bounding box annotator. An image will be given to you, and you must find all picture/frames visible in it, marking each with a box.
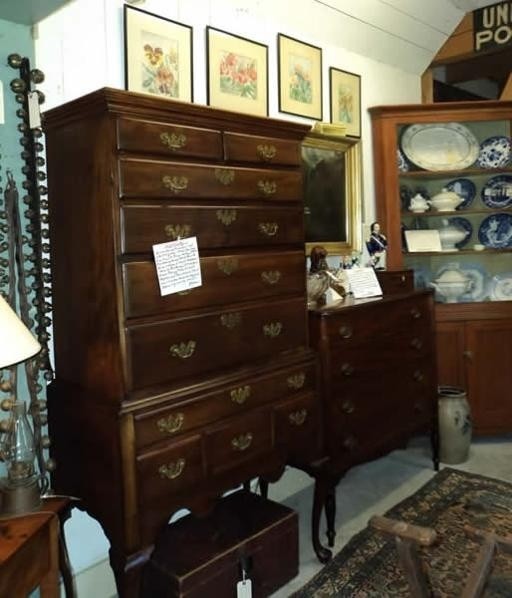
[204,22,271,118]
[121,1,196,105]
[328,65,364,140]
[304,127,365,255]
[275,30,325,123]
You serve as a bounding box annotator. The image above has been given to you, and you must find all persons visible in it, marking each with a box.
[365,221,389,271]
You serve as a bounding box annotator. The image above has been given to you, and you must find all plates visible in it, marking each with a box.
[395,122,512,303]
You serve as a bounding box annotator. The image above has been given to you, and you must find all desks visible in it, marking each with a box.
[1,493,76,598]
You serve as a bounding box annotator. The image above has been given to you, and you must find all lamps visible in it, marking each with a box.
[0,293,45,372]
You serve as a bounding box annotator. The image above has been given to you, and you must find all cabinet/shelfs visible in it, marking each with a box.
[367,98,512,441]
[37,85,317,402]
[258,284,444,549]
[45,350,334,596]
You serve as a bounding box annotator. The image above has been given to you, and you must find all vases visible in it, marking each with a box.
[436,385,476,467]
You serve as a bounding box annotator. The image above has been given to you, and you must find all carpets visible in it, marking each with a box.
[287,465,512,597]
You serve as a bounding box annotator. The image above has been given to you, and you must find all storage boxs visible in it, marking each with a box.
[371,266,417,296]
